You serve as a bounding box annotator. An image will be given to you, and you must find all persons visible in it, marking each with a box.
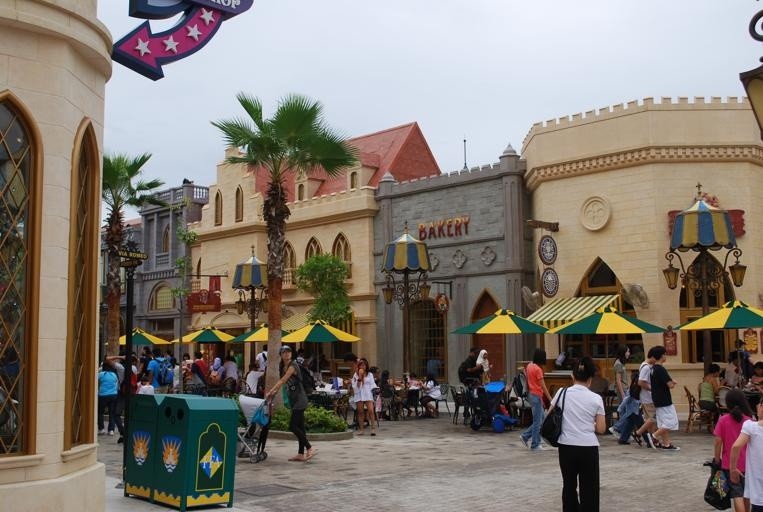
[609,344,680,452]
[697,338,763,512]
[98,351,127,443]
[183,352,238,396]
[456,345,482,418]
[371,368,441,420]
[475,349,495,382]
[132,346,180,394]
[343,358,377,435]
[295,349,328,385]
[520,348,553,451]
[547,356,606,512]
[266,346,319,460]
[246,346,268,396]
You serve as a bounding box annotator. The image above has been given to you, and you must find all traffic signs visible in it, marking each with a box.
[118,258,144,269]
[114,249,150,260]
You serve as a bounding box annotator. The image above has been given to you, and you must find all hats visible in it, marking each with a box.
[470,347,480,353]
[736,339,746,346]
[280,345,292,353]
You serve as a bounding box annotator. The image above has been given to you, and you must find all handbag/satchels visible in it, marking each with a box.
[288,361,315,394]
[513,372,527,397]
[540,405,562,447]
[458,362,466,382]
[630,371,641,400]
[703,458,731,510]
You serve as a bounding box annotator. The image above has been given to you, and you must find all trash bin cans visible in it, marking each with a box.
[124,394,239,512]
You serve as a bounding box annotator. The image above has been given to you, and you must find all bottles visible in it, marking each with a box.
[334,377,339,390]
[555,351,569,367]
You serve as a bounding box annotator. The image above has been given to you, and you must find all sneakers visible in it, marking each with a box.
[118,437,123,443]
[609,426,680,450]
[520,434,529,448]
[532,446,545,450]
[98,429,114,436]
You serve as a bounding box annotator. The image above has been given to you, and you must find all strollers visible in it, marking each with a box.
[235,390,278,463]
[462,374,519,432]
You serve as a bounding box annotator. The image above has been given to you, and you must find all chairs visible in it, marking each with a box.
[512,384,714,436]
[184,382,477,432]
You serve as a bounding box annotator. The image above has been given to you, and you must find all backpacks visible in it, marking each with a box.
[210,365,222,385]
[151,358,174,385]
[121,367,137,395]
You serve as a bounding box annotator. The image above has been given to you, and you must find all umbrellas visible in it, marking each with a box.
[101,318,362,370]
[450,309,548,381]
[546,304,668,396]
[674,300,762,389]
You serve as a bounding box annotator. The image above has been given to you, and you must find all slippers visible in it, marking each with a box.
[288,449,318,462]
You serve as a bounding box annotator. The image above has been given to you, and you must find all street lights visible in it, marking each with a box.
[657,246,750,434]
[382,270,431,387]
[233,288,270,373]
